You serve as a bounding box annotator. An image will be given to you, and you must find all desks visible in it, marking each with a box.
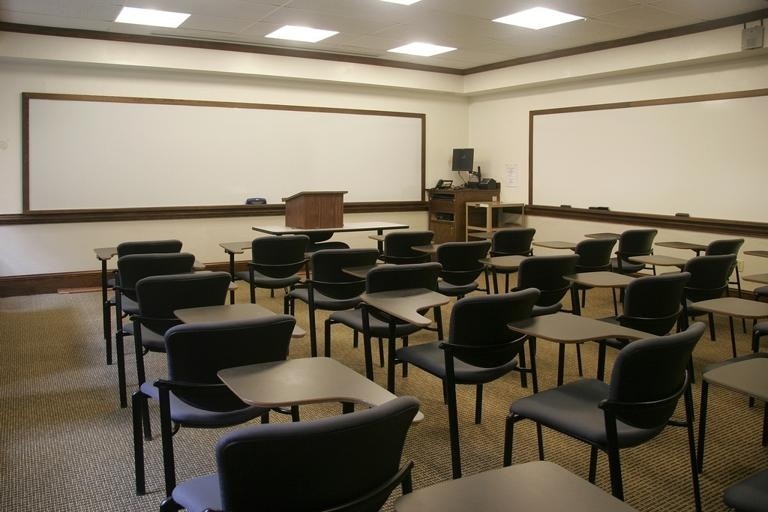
[368,232,385,241]
[218,238,254,278]
[93,245,111,265]
[477,253,531,274]
[338,261,395,281]
[531,238,580,250]
[626,253,690,269]
[389,458,638,512]
[172,302,308,342]
[562,269,641,290]
[216,355,426,430]
[740,248,768,257]
[740,270,768,286]
[409,243,446,253]
[251,188,410,235]
[654,240,710,260]
[581,232,623,240]
[694,356,768,476]
[687,295,768,322]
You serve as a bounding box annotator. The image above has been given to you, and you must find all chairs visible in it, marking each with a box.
[378,229,435,265]
[282,244,380,360]
[434,238,493,295]
[130,313,302,503]
[132,270,233,440]
[707,238,747,342]
[109,251,196,410]
[720,469,768,512]
[170,394,422,512]
[683,254,737,301]
[104,238,184,366]
[323,262,446,383]
[588,270,693,386]
[572,238,617,271]
[502,320,708,506]
[610,228,657,275]
[230,235,310,308]
[511,253,582,316]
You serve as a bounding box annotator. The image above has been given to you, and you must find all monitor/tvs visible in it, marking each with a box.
[452,148,474,171]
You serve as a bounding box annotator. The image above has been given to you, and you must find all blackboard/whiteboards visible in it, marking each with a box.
[20,92,426,215]
[528,89,768,228]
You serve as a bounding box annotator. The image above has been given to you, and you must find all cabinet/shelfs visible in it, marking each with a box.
[466,200,526,244]
[425,186,500,244]
